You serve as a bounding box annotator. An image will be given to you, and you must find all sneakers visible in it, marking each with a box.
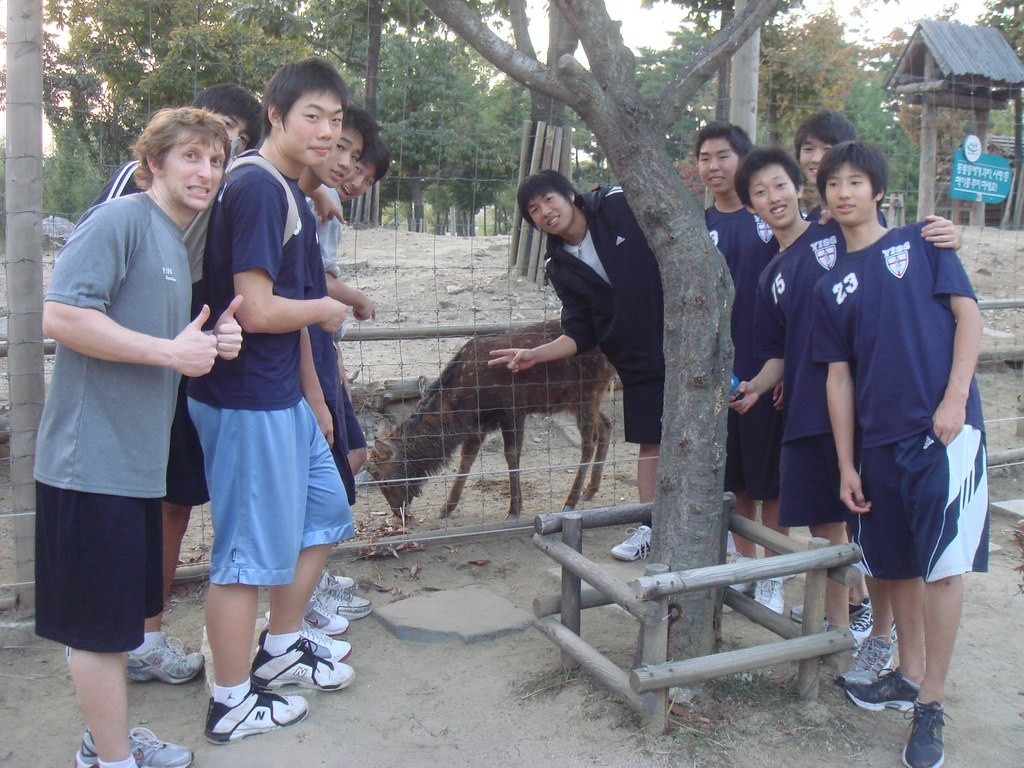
[304,599,350,636]
[726,552,757,597]
[125,635,203,685]
[844,635,892,685]
[202,683,309,745]
[311,572,373,619]
[259,618,353,662]
[77,728,194,767]
[847,667,921,711]
[790,602,804,622]
[251,637,355,691]
[902,695,945,768]
[753,574,784,615]
[611,524,651,562]
[848,597,897,640]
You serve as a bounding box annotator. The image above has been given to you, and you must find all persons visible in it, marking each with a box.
[33,58,390,768]
[696,110,990,768]
[488,169,667,562]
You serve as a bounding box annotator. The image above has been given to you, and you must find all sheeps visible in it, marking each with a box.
[362,320,614,526]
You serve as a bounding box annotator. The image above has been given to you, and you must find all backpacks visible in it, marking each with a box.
[183,147,296,284]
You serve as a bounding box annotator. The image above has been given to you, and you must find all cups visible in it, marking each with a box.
[729,373,745,402]
